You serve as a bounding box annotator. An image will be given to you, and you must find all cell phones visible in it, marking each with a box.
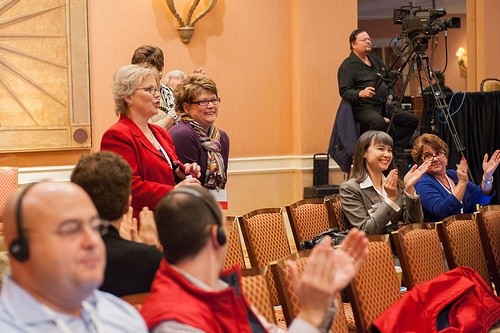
[173,161,197,180]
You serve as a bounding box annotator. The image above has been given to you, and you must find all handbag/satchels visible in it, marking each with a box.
[306,228,350,303]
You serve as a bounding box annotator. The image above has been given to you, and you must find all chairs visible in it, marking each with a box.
[124,193,500,333]
[0,165,21,238]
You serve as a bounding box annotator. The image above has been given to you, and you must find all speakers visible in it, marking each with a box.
[304,183,340,198]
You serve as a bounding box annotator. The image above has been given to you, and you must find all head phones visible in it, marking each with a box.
[173,188,227,246]
[9,183,36,261]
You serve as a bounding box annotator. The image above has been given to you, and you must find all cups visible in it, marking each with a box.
[395,265,403,289]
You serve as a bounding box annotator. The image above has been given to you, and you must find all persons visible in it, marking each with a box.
[142,183,371,333]
[411,126,500,222]
[98,63,201,233]
[339,130,431,236]
[168,72,230,192]
[420,71,453,96]
[337,28,420,156]
[69,151,165,297]
[132,46,177,132]
[0,179,149,333]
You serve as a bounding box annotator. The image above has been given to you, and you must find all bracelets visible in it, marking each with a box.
[482,175,494,184]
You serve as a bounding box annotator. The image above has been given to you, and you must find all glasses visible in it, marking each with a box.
[190,98,220,106]
[421,152,445,161]
[133,88,157,94]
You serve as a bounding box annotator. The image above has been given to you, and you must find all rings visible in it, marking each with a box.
[462,170,464,172]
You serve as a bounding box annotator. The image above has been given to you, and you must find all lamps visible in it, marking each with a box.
[456,47,468,69]
[166,0,218,44]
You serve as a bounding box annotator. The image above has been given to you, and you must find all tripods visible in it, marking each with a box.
[385,40,476,184]
